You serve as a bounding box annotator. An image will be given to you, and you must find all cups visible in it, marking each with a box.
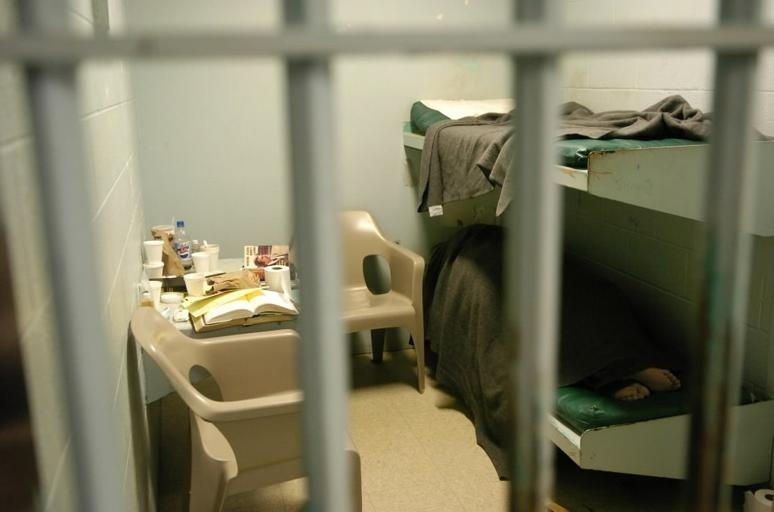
[183,244,219,298]
[143,239,164,309]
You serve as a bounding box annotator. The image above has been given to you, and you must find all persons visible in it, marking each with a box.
[427,242,681,402]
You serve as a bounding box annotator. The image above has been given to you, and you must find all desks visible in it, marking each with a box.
[143,258,303,512]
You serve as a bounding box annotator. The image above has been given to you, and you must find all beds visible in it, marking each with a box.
[399,98,773,488]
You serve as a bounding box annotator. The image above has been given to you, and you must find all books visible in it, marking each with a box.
[187,245,300,333]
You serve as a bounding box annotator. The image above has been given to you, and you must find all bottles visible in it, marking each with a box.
[174,220,199,270]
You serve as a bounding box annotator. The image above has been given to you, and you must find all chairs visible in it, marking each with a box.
[130,305,362,512]
[340,211,425,394]
